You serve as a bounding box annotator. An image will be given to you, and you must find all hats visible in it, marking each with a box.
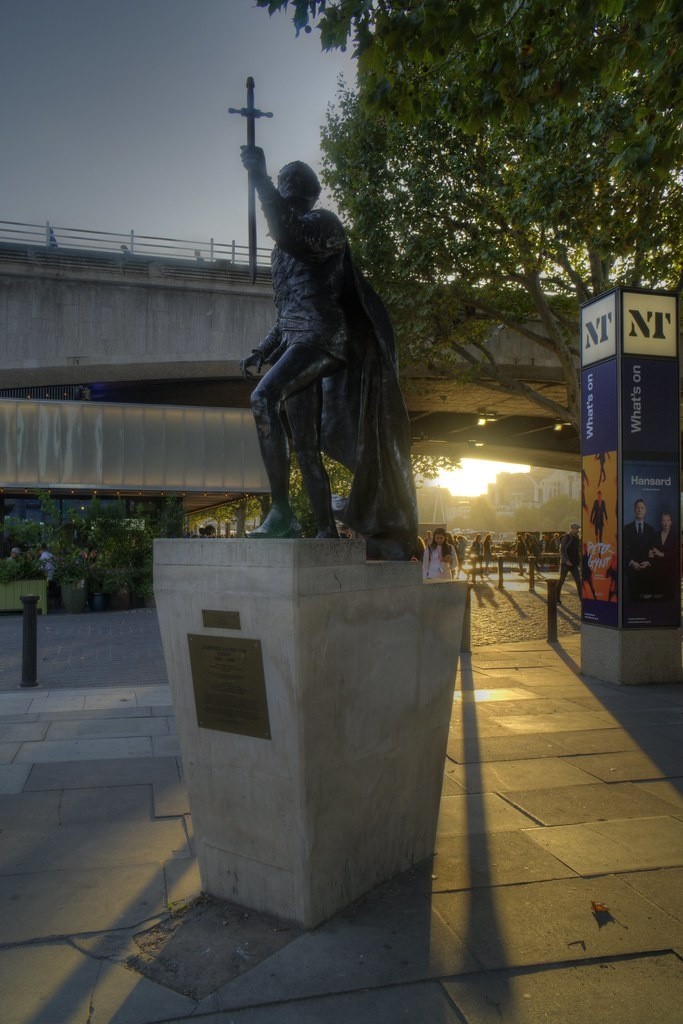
[570,524,581,530]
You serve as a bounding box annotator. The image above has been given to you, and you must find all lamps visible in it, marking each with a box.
[553,416,563,432]
[475,410,487,426]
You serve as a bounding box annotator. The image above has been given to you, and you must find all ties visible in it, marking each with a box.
[637,523,642,537]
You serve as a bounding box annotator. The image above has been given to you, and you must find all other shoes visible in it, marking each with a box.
[557,598,563,605]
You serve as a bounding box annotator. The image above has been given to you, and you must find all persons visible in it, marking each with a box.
[582,450,678,601]
[413,522,582,604]
[239,146,349,541]
[38,543,56,593]
[205,526,216,539]
[8,548,20,561]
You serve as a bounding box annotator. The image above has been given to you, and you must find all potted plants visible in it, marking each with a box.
[0,485,190,614]
